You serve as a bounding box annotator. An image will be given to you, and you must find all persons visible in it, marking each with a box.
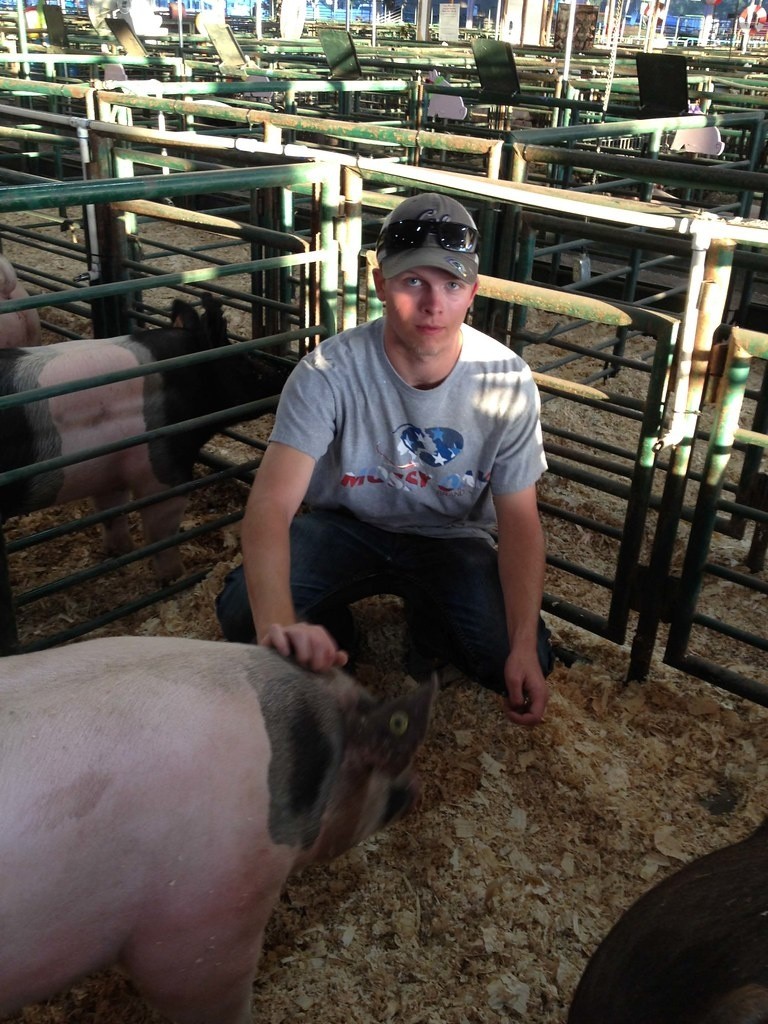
[216,191,556,727]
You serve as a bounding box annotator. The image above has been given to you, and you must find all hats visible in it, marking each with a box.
[377,193,479,284]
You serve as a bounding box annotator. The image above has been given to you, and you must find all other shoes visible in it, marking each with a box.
[404,607,435,680]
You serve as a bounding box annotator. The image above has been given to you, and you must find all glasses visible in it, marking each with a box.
[377,218,479,253]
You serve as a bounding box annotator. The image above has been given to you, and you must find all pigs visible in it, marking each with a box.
[565,811,768,1023]
[0,252,295,583]
[1,634,438,1024]
